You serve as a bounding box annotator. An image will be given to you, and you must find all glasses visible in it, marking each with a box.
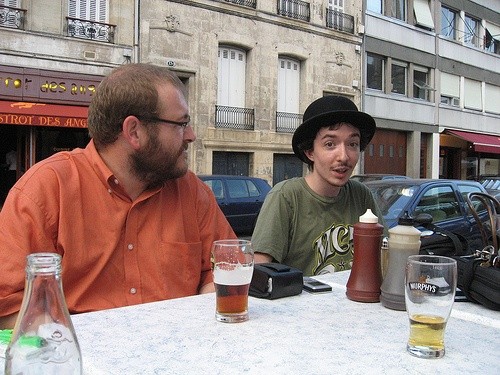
[120,115,191,131]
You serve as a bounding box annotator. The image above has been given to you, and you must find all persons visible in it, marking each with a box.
[245,95,389,277]
[0,63,248,330]
[6,145,17,187]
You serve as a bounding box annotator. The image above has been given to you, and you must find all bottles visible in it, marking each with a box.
[382,236,389,279]
[379,210,422,312]
[345,207,385,303]
[5,251,83,375]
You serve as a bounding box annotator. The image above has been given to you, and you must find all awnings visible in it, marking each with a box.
[444,129,500,154]
[0,100,88,128]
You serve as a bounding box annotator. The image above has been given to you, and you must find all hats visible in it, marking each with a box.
[292,95,376,165]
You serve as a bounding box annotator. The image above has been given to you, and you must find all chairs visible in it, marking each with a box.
[466,191,500,260]
[220,182,230,197]
[421,196,446,221]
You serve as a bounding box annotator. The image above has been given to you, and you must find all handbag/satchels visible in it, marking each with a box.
[444,245,500,311]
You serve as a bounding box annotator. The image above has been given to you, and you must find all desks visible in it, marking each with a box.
[0,264,500,375]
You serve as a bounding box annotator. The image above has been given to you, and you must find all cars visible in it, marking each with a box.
[348,174,410,183]
[361,178,496,247]
[195,174,273,241]
[479,177,500,214]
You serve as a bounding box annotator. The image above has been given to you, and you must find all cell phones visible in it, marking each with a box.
[303,276,332,292]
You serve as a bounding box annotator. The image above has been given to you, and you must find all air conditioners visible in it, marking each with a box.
[451,98,460,106]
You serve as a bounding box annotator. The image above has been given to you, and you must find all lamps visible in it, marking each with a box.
[355,45,360,54]
[357,25,364,33]
[122,48,132,65]
[352,80,359,87]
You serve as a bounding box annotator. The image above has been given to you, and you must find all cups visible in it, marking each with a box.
[210,239,255,324]
[404,254,458,360]
